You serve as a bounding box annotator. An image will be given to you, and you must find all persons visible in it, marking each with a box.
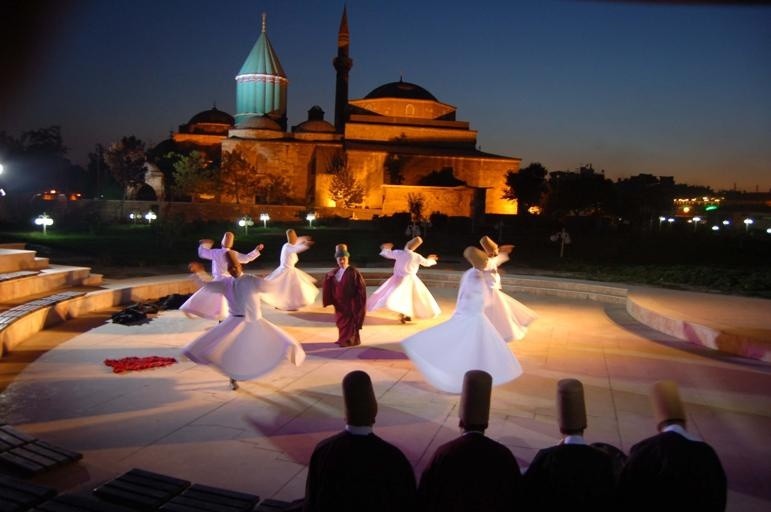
[396,245,525,395]
[178,231,264,323]
[366,235,443,324]
[613,379,726,511]
[416,369,522,512]
[304,370,416,511]
[480,235,539,344]
[260,229,319,311]
[182,250,305,390]
[322,244,366,347]
[520,378,622,511]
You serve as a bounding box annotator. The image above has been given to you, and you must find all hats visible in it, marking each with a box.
[226,251,240,269]
[649,382,686,425]
[221,231,235,248]
[557,378,586,430]
[459,370,492,427]
[334,244,350,258]
[287,229,297,243]
[479,236,498,254]
[463,245,487,267]
[407,236,423,251]
[343,371,377,423]
